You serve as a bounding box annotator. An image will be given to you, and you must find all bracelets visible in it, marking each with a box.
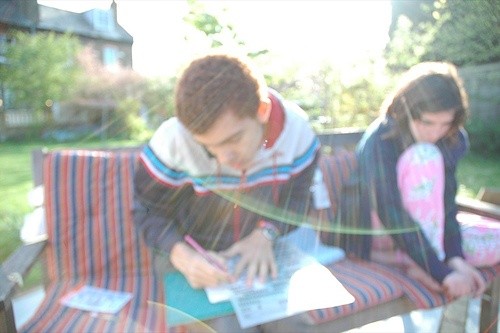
[253,224,277,242]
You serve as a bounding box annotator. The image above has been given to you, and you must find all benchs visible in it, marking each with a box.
[1,128,500,333]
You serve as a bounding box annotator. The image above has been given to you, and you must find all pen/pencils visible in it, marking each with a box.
[184,235,219,265]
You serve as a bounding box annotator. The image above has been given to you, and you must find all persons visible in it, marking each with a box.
[327,60,487,302]
[129,54,322,333]
